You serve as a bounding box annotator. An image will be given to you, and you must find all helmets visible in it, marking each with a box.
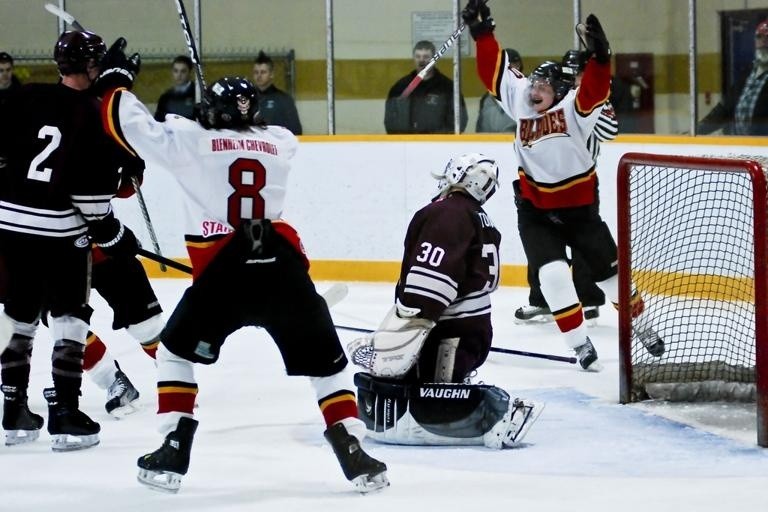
[430,152,499,206]
[561,49,583,73]
[196,76,264,130]
[527,60,576,99]
[54,30,106,70]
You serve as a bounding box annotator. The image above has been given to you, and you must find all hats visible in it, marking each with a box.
[506,48,521,63]
[753,21,767,37]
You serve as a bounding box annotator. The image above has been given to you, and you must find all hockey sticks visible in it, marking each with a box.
[44,4,167,272]
[74,233,193,275]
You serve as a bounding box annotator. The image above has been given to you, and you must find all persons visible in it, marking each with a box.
[475,48,523,133]
[560,50,619,165]
[82,157,169,414]
[95,37,387,483]
[680,21,768,137]
[252,50,302,136]
[346,149,547,450]
[153,55,199,123]
[0,51,23,91]
[0,29,141,436]
[384,40,468,134]
[461,0,665,369]
[514,245,605,320]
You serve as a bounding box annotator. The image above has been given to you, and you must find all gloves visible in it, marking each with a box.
[90,215,142,263]
[576,13,610,65]
[462,0,495,41]
[96,37,141,98]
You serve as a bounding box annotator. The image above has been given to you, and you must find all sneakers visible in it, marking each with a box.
[573,336,599,369]
[634,328,665,357]
[323,423,387,481]
[105,359,139,413]
[502,398,545,449]
[137,416,199,475]
[583,306,600,320]
[514,304,553,320]
[43,389,101,435]
[1,384,44,430]
